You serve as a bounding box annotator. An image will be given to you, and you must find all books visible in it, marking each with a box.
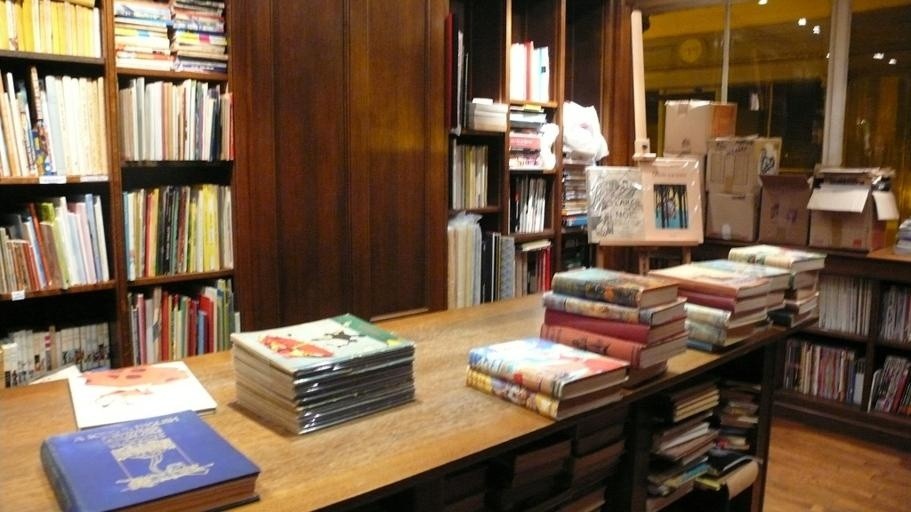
[646,243,827,355]
[35,407,263,512]
[784,340,827,398]
[828,218,911,421]
[61,357,218,431]
[225,312,418,439]
[1,2,111,391]
[387,235,828,512]
[467,265,690,422]
[444,378,762,512]
[440,39,601,313]
[113,1,236,366]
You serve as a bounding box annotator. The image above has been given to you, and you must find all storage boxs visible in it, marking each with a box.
[662,98,901,253]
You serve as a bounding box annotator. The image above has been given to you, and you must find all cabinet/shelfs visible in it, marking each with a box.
[1,0,123,384]
[0,289,819,512]
[685,236,911,453]
[447,0,615,311]
[109,2,237,390]
